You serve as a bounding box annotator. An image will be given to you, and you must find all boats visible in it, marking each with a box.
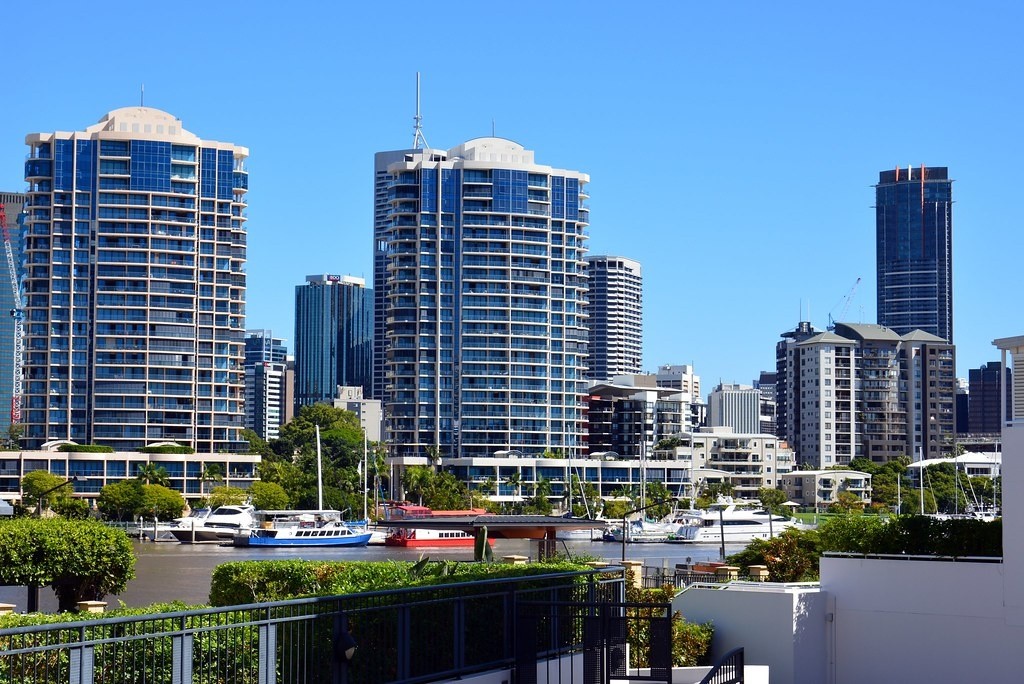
[893,442,1001,523]
[137,425,498,548]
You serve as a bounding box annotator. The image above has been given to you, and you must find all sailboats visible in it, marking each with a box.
[537,424,801,543]
